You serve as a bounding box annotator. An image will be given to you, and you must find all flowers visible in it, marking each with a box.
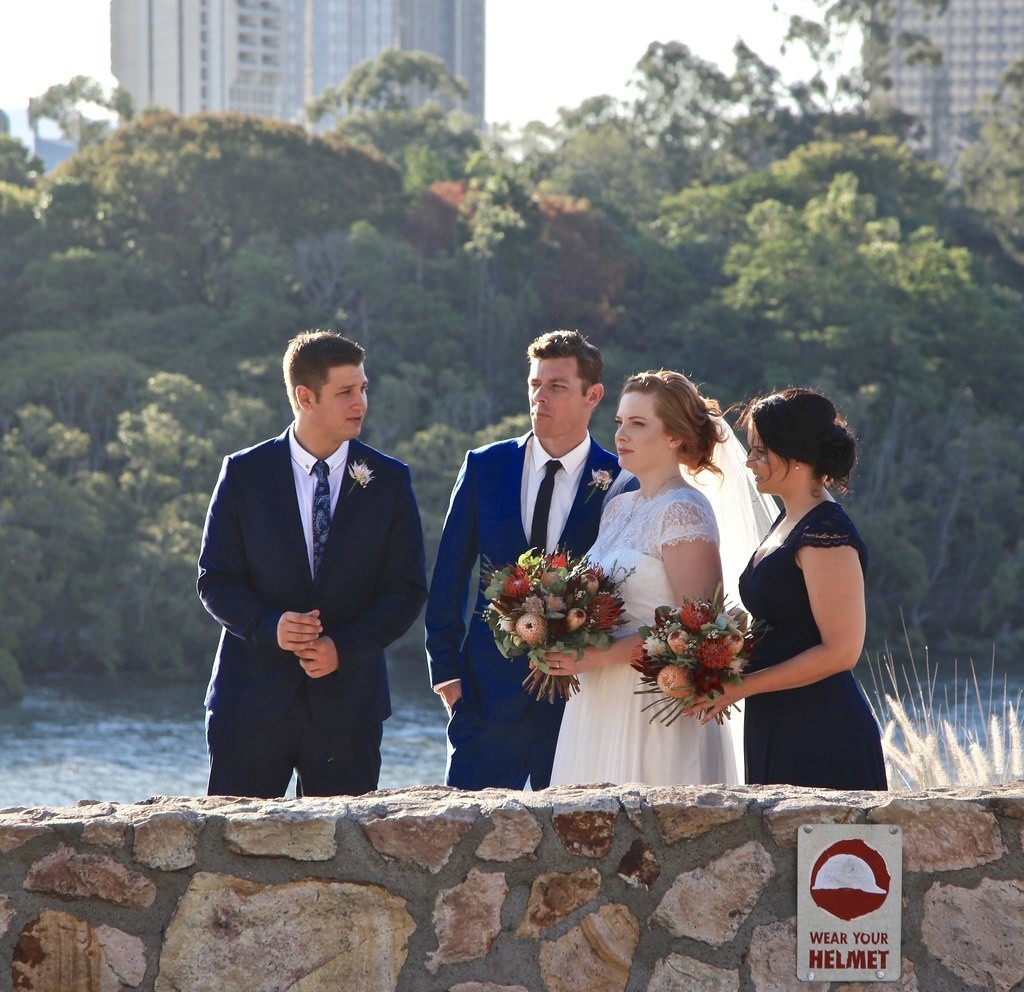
[346,459,375,496]
[629,582,774,727]
[585,469,613,504]
[472,541,636,705]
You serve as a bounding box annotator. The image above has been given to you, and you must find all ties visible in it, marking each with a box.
[312,459,332,583]
[529,459,563,560]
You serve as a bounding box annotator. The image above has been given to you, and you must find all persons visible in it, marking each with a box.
[681,387,890,790]
[422,328,641,791]
[195,329,429,799]
[527,368,743,788]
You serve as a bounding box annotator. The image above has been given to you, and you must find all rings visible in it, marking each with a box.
[556,661,560,669]
